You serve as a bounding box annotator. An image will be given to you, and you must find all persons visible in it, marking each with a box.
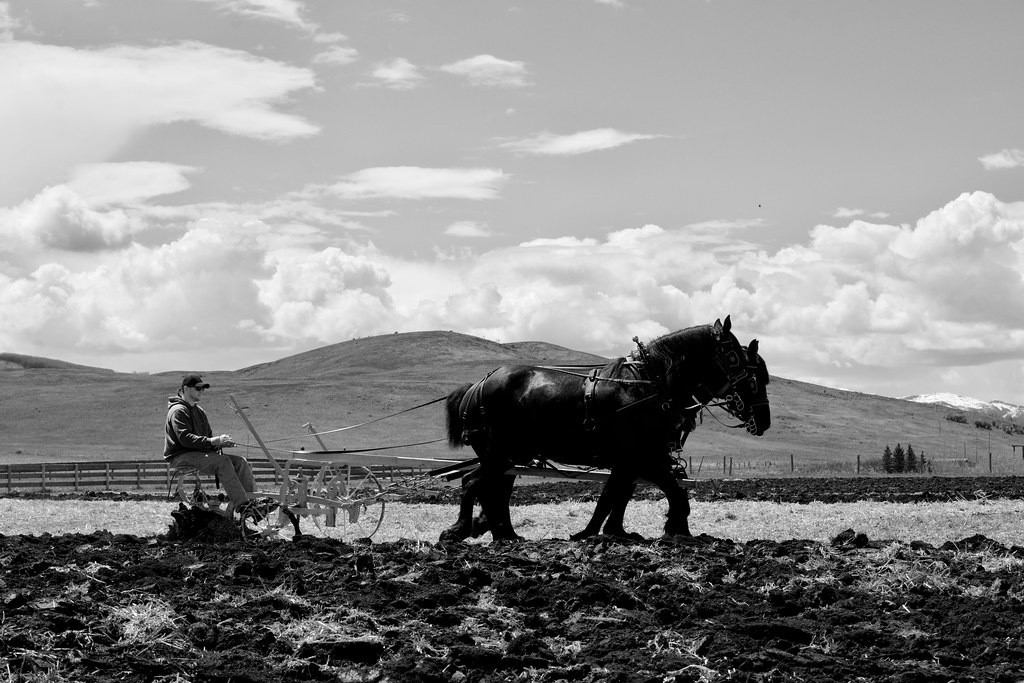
[163,375,279,522]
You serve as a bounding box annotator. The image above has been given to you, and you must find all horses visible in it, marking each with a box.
[438,314,772,543]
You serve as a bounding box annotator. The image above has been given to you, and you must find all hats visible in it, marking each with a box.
[182,375,210,389]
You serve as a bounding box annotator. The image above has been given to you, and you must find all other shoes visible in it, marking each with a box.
[241,496,279,521]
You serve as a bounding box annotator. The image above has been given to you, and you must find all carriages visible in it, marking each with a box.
[165,316,773,539]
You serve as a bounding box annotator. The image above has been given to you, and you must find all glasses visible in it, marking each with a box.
[194,386,204,391]
